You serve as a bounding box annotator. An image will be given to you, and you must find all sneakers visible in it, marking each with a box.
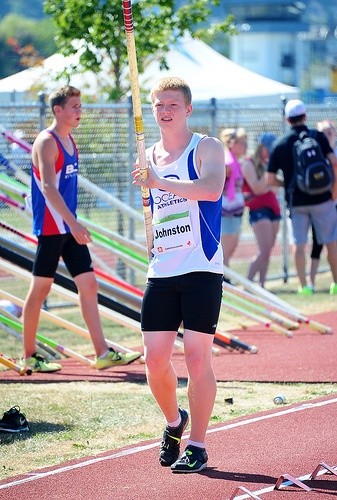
[96,348,142,371]
[23,353,61,373]
[0,405,29,433]
[297,285,312,296]
[171,445,208,473]
[330,282,337,294]
[159,407,189,467]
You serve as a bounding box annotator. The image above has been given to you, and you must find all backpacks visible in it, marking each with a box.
[285,128,333,194]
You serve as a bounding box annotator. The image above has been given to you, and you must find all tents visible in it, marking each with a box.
[0,13,304,212]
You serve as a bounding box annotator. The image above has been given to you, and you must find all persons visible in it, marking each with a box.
[130,77,227,475]
[22,84,143,374]
[222,99,337,296]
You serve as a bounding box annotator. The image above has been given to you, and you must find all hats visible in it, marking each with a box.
[285,100,305,118]
[256,133,276,154]
